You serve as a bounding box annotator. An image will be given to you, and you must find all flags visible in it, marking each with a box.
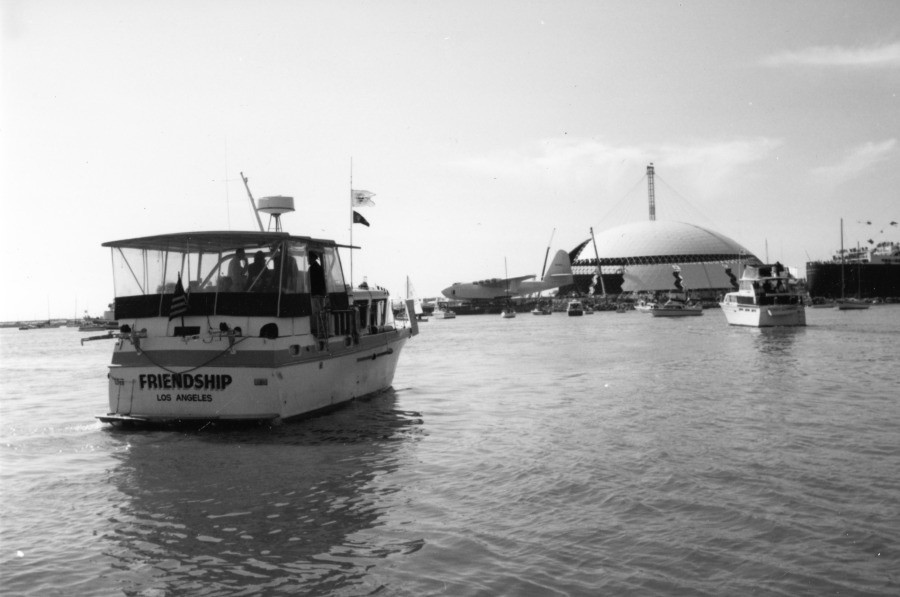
[351,189,376,227]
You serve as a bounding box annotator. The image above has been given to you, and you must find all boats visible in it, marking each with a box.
[391,276,436,322]
[531,308,543,315]
[718,263,806,326]
[80,172,435,428]
[104,321,119,329]
[649,300,702,317]
[634,305,653,313]
[432,305,455,319]
[79,321,105,331]
[19,327,27,330]
[501,305,515,318]
[835,301,869,309]
[566,302,583,316]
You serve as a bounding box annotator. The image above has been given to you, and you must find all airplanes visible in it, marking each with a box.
[440,238,594,300]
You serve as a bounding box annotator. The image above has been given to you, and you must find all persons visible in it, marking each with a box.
[227,241,325,338]
[754,279,786,304]
